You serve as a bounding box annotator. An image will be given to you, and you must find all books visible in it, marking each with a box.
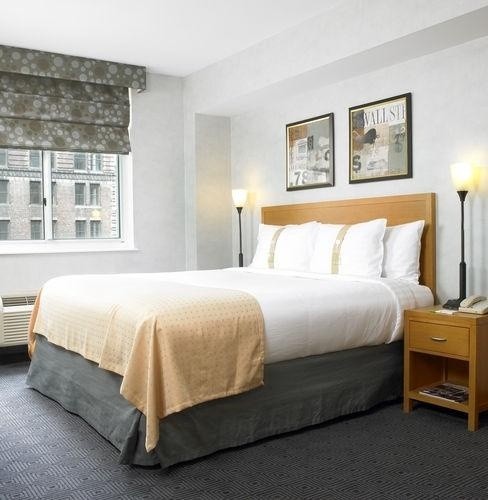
[419,382,469,403]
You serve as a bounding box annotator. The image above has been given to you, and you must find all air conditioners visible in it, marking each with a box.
[0,291,38,347]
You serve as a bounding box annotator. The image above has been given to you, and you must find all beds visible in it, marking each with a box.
[26,191,436,474]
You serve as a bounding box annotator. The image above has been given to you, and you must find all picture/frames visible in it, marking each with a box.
[285,112,335,191]
[349,92,413,184]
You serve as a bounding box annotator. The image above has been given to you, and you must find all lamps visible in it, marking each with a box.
[443,170,480,308]
[232,189,248,267]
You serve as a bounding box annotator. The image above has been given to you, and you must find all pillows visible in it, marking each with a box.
[305,217,388,280]
[382,220,426,286]
[248,220,320,273]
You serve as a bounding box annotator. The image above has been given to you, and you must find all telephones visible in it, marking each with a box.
[459,296,488,314]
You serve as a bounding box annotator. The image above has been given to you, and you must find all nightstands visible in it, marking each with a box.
[402,304,488,432]
